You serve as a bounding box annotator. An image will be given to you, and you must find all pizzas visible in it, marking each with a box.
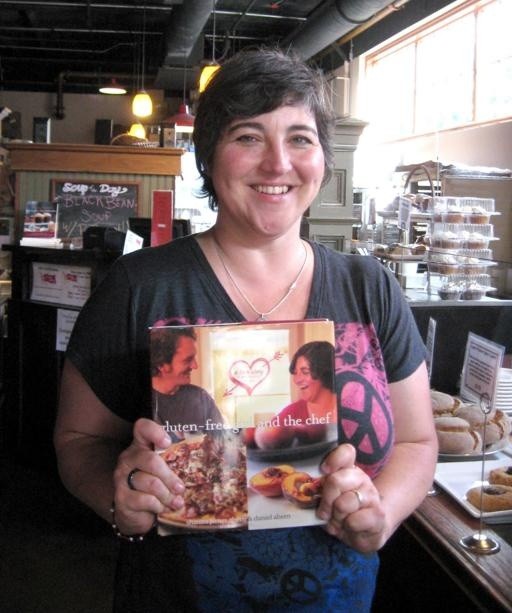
[160,432,247,524]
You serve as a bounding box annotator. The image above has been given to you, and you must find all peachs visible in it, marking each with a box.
[242,425,327,449]
[251,464,296,498]
[281,472,322,508]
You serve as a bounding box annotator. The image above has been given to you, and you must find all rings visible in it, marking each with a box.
[127,469,141,491]
[354,489,364,508]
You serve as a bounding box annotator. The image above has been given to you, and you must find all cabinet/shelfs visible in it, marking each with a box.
[377,166,512,298]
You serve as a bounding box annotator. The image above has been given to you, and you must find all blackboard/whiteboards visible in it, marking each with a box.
[50,178,143,244]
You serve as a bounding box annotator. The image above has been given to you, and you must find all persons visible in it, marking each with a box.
[272,341,339,428]
[148,326,227,444]
[53,43,439,613]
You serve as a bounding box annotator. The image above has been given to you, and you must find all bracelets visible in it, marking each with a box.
[109,498,145,542]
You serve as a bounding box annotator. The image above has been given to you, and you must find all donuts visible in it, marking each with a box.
[489,464,511,485]
[466,485,512,511]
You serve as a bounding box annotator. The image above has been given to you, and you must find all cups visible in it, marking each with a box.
[387,261,418,277]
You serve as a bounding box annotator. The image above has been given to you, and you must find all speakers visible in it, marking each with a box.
[95,119,112,145]
[33,117,51,144]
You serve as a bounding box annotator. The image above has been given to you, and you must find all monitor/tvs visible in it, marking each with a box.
[128,217,191,248]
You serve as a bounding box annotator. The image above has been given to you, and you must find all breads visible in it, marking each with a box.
[48,221,54,231]
[454,404,512,451]
[403,193,433,208]
[434,417,483,454]
[372,243,426,255]
[430,389,465,419]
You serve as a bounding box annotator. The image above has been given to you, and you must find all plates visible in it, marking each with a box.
[158,512,248,531]
[438,445,510,462]
[460,368,512,416]
[433,459,512,525]
[247,422,338,463]
[410,212,431,218]
[377,209,399,217]
[374,250,427,261]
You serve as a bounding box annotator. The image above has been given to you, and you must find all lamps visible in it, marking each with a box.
[99,78,127,94]
[127,0,223,140]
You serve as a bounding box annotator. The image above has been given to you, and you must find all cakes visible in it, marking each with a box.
[423,201,489,301]
[33,212,52,223]
